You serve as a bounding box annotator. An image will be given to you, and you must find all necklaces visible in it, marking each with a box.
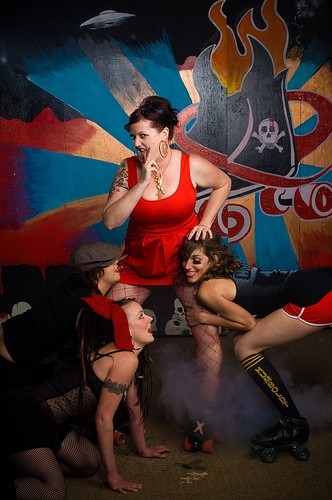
[151,150,172,195]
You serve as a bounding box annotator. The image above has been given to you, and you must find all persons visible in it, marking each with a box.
[0,293,173,500]
[105,96,231,453]
[0,242,131,377]
[180,230,332,464]
